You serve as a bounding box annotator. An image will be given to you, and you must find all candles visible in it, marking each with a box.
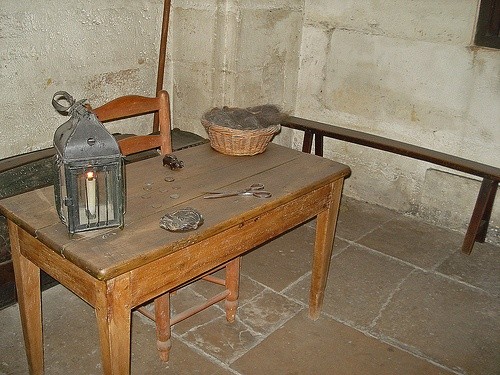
[85,172,96,217]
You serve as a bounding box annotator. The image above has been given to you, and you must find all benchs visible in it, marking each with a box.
[280,115,500,256]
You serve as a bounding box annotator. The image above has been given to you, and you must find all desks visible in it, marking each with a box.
[1,142,351,375]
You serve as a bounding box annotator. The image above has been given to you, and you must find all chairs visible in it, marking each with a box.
[84,90,241,362]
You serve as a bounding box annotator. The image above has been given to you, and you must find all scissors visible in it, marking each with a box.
[201,182,273,202]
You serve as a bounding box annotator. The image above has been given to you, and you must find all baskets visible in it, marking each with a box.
[201,112,281,157]
